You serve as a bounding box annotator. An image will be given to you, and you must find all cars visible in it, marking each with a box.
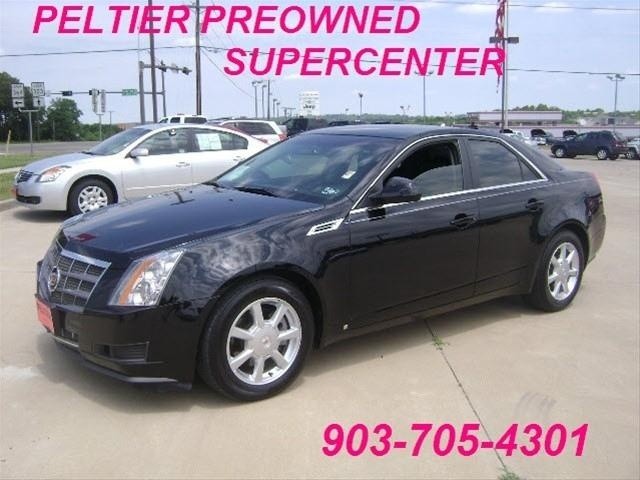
[13,124,269,217]
[535,130,640,160]
[35,123,607,402]
[156,112,286,145]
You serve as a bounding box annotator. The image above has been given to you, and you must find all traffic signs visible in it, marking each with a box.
[32,82,44,107]
[12,84,25,107]
[123,90,137,96]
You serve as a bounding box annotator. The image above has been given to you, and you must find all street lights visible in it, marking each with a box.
[415,71,432,124]
[251,80,296,117]
[609,73,627,132]
[359,94,363,121]
[489,37,519,134]
